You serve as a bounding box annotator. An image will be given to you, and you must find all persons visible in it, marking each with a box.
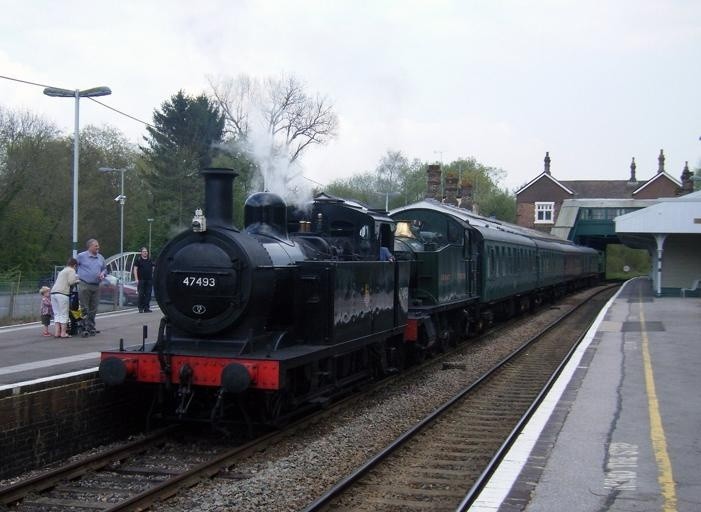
[39,286,52,337]
[76,238,107,333]
[50,257,81,338]
[380,247,396,264]
[133,246,153,313]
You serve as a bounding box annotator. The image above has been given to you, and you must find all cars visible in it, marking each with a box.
[127,278,155,298]
[38,276,58,290]
[72,273,139,306]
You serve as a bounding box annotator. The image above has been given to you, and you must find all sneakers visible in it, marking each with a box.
[139,310,143,313]
[54,333,72,338]
[144,309,152,313]
[43,332,51,336]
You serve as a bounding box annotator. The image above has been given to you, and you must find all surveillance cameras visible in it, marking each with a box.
[114,195,121,202]
[121,196,128,199]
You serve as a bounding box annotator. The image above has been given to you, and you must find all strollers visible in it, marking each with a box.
[65,283,96,338]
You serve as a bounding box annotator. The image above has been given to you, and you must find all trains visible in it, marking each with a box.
[99,165,601,437]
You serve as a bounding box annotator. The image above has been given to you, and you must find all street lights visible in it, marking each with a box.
[433,149,449,203]
[147,218,155,260]
[44,86,113,291]
[372,191,401,212]
[99,167,128,309]
